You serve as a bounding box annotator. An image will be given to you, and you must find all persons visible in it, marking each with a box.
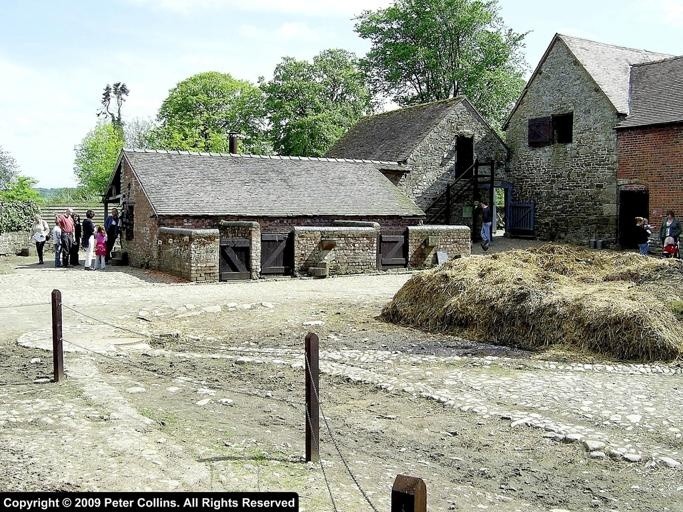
[51,208,121,270]
[474,200,491,251]
[28,214,50,265]
[659,210,682,259]
[642,218,656,244]
[634,217,650,256]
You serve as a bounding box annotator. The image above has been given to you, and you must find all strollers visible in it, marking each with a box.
[657,236,680,260]
